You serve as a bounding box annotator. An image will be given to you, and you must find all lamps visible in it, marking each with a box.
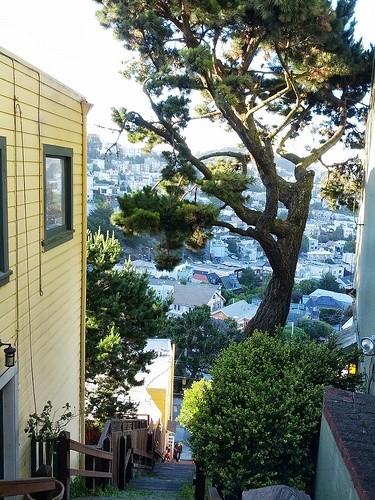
[360,335,374,356]
[0,339,17,368]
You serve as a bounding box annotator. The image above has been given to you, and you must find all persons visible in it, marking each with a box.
[174,441,182,464]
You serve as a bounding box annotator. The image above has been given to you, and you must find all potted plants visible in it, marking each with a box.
[24,400,99,500]
